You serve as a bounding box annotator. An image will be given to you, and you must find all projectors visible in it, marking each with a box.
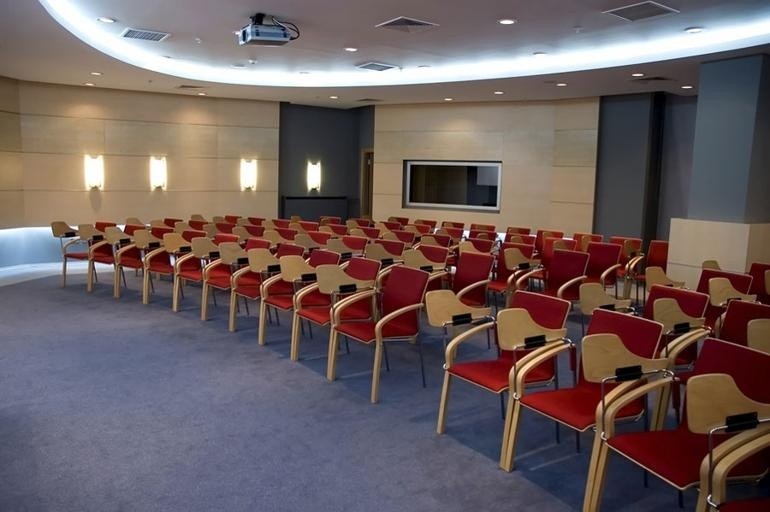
[238,23,291,46]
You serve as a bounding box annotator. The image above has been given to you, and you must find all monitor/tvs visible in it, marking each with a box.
[405,161,502,211]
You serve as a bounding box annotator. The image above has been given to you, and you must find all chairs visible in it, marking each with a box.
[51,215,438,404]
[402,219,770,512]
